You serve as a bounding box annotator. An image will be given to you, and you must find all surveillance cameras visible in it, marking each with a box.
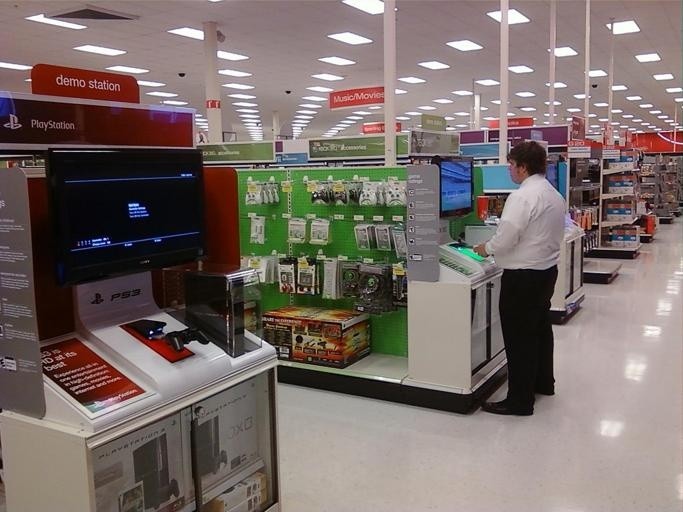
[285,90,291,94]
[178,72,186,77]
[591,83,598,88]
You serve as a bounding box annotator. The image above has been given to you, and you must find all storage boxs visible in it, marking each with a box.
[260,305,373,370]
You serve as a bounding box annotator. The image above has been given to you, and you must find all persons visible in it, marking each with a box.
[473,140,566,416]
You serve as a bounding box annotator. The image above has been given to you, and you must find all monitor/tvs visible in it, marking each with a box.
[431,155,473,218]
[47,147,208,286]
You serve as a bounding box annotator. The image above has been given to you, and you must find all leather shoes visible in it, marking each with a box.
[481,401,533,415]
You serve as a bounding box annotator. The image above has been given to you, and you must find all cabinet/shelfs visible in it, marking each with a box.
[600,152,683,256]
